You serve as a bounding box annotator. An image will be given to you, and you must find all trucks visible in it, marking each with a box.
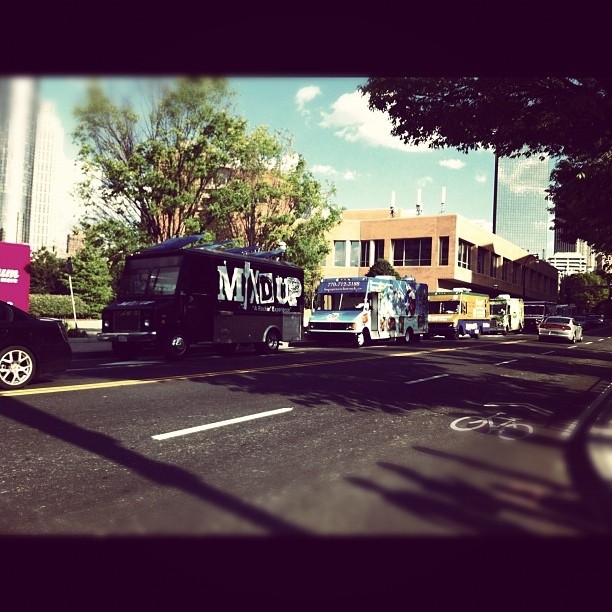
[557,305,572,316]
[96,233,304,359]
[482,294,524,334]
[304,275,428,347]
[423,292,491,340]
[524,302,557,333]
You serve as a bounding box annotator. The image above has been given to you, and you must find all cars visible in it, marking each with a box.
[538,316,583,344]
[0,301,72,391]
